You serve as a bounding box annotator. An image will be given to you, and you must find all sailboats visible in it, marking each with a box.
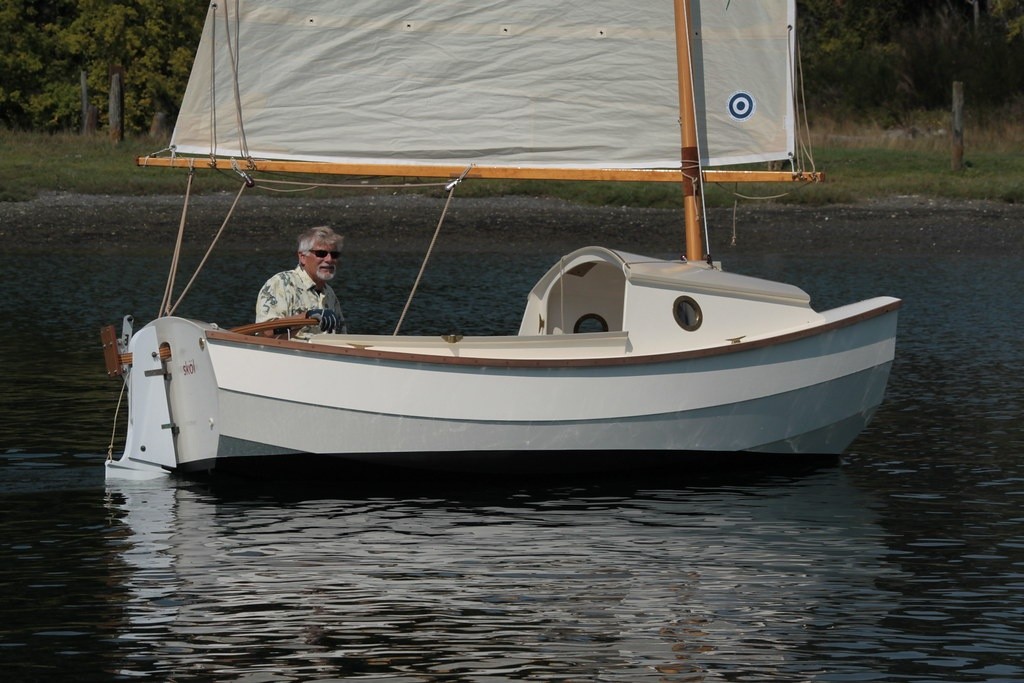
[100,2,902,482]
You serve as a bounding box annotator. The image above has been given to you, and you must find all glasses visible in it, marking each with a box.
[309,249,340,259]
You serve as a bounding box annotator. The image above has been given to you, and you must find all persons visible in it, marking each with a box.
[254,225,347,339]
